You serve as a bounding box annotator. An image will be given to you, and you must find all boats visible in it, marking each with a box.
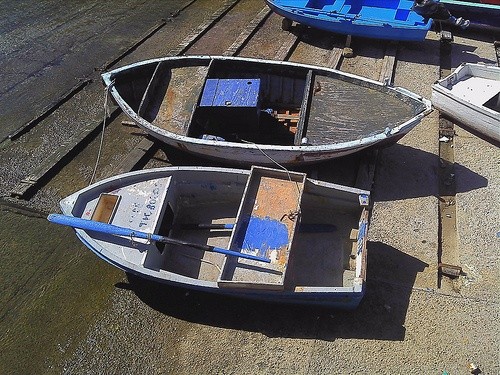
[265,1,471,42]
[102,55,435,163]
[442,0,500,33]
[59,164,374,308]
[431,61,499,141]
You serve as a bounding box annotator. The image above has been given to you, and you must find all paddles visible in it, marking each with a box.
[279,3,418,27]
[47,210,271,263]
[159,222,339,232]
